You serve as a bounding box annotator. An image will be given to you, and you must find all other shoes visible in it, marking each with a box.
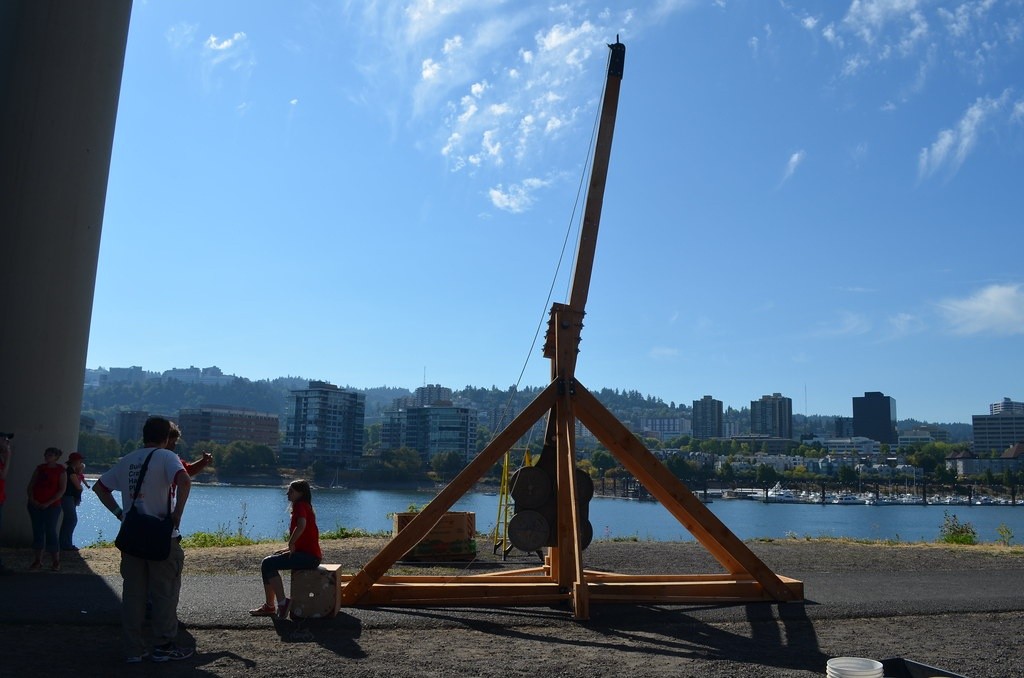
[51,563,61,571]
[29,561,44,570]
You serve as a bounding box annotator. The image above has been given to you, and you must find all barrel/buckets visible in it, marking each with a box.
[826,657,884,678]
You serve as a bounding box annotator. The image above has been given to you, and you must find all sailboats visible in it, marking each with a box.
[330,465,347,490]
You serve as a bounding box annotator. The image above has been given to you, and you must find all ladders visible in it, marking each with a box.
[493,448,548,563]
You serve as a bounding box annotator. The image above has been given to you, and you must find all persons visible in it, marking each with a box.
[26,447,90,570]
[249,480,322,620]
[0,432,14,520]
[92,416,214,662]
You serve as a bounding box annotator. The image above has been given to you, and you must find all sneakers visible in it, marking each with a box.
[127,649,149,663]
[152,643,194,662]
[277,597,292,622]
[249,603,276,616]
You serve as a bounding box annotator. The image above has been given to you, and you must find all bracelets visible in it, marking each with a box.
[112,506,120,515]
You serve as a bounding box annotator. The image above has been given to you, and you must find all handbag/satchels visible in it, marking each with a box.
[115,507,174,563]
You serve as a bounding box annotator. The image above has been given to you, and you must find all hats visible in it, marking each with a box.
[0,432,14,440]
[69,453,87,462]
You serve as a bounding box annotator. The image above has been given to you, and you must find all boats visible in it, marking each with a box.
[747,481,1024,505]
[485,492,497,496]
[721,491,740,498]
